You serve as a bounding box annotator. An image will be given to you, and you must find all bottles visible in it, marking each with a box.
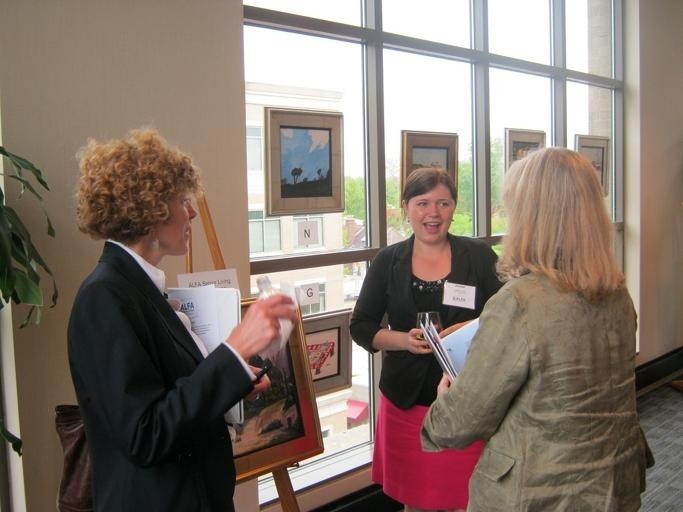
[256,276,279,297]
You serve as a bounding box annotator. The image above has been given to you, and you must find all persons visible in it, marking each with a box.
[418,146,654,512]
[68,129,300,512]
[349,168,507,511]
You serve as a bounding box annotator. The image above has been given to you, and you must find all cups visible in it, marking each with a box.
[417,312,445,348]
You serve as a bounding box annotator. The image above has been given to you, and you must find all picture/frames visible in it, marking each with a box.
[261,106,344,217]
[575,134,610,197]
[503,127,547,178]
[400,130,458,210]
[301,306,353,398]
[205,298,325,487]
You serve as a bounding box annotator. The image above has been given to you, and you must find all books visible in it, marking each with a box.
[420,317,479,383]
[164,285,244,425]
